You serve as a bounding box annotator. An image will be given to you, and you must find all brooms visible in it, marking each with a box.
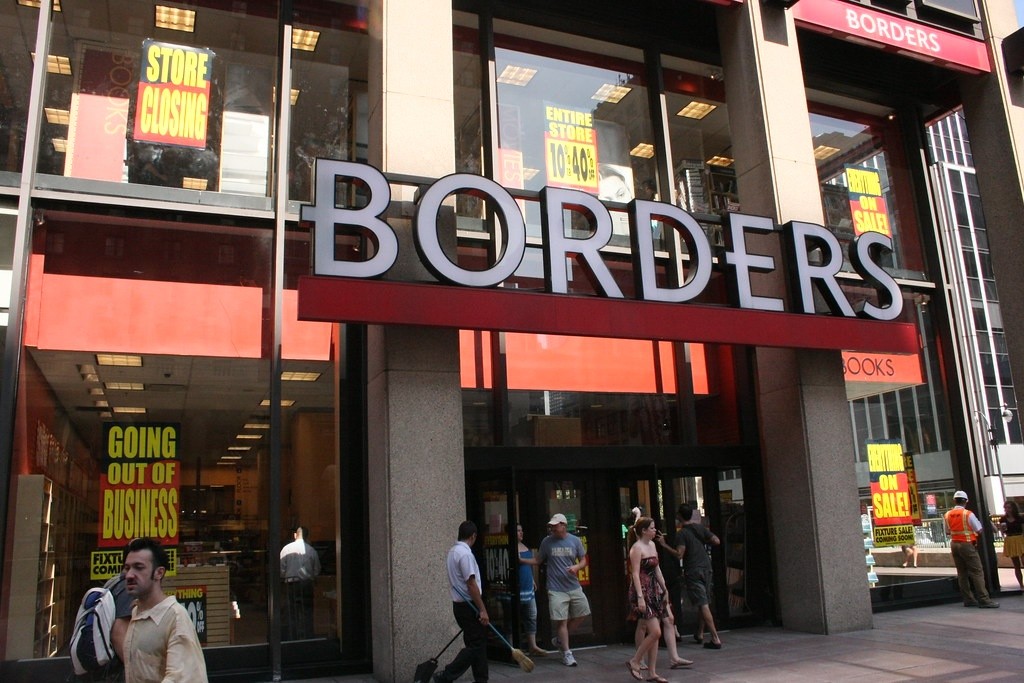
[452,585,535,673]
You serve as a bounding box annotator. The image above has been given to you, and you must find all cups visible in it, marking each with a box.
[1001,522,1007,531]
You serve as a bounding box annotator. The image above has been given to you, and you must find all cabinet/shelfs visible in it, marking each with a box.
[161,565,230,647]
[700,165,739,244]
[5,474,97,660]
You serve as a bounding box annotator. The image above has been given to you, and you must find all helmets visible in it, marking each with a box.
[952,491,968,502]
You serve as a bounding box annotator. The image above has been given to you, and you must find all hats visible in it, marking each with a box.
[547,513,567,525]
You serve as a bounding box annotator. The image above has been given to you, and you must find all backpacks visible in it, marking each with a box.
[70,575,127,676]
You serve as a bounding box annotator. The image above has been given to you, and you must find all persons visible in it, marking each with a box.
[999,500,1024,588]
[518,514,591,666]
[517,521,547,657]
[944,491,1000,609]
[657,503,721,649]
[641,180,656,200]
[623,506,693,683]
[280,527,322,640]
[104,537,209,683]
[434,520,489,683]
[598,166,632,203]
[902,544,918,567]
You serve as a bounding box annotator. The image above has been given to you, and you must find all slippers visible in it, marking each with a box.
[645,674,668,683]
[693,632,703,643]
[704,640,721,649]
[625,660,641,680]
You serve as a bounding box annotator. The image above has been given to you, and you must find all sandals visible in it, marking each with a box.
[639,660,648,670]
[670,658,693,669]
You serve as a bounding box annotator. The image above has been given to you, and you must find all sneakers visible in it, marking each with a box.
[551,636,561,652]
[561,651,577,665]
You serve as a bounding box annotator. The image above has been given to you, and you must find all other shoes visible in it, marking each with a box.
[529,647,546,656]
[434,670,452,683]
[968,600,978,607]
[979,601,1000,608]
[675,633,682,642]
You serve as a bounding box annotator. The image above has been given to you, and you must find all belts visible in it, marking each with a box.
[285,576,300,583]
